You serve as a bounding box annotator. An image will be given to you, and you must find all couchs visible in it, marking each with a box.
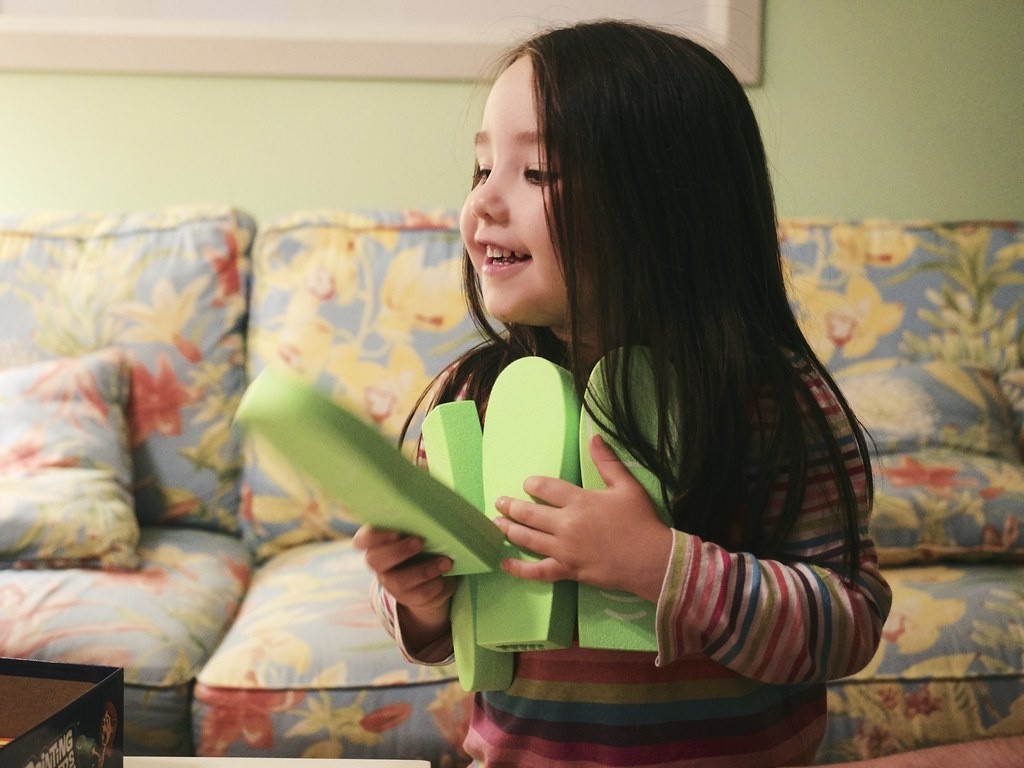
[0,208,1024,768]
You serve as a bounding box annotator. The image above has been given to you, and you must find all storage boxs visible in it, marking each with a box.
[1,657,124,768]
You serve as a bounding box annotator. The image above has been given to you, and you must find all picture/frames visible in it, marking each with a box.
[1,0,765,88]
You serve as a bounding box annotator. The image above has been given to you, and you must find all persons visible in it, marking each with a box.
[352,15,892,768]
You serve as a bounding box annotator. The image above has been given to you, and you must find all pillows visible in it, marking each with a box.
[832,357,1024,565]
[0,348,143,572]
